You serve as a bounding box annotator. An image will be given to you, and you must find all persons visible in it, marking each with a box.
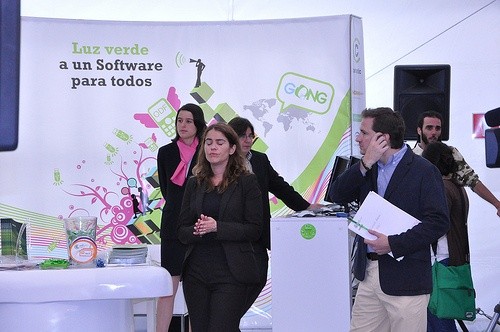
[155,104,208,332]
[228,117,329,322]
[410,110,500,332]
[331,107,450,332]
[177,123,263,332]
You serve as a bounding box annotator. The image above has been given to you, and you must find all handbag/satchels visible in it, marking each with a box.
[428,261,476,321]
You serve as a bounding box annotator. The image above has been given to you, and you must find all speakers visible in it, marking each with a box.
[394,64,451,141]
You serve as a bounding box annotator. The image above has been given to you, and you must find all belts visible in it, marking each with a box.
[367,252,379,260]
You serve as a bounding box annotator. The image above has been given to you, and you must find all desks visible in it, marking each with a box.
[270,216,353,331]
[0,264,173,332]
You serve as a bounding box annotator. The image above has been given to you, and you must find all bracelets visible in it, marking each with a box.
[361,157,371,170]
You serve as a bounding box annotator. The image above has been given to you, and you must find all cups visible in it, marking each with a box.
[64,216,97,267]
